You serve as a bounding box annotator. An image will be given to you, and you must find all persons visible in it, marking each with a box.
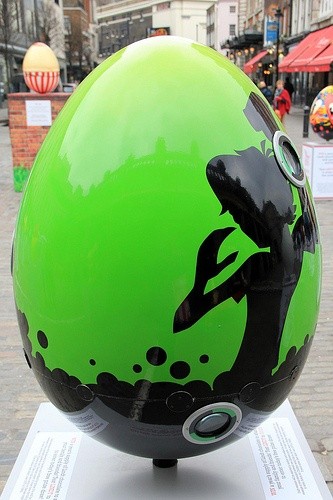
[273,80,292,120]
[257,81,271,101]
[283,77,295,116]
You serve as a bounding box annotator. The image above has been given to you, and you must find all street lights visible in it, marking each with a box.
[228,45,283,107]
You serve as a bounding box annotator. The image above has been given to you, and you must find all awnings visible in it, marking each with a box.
[277,24,332,73]
[242,50,269,74]
